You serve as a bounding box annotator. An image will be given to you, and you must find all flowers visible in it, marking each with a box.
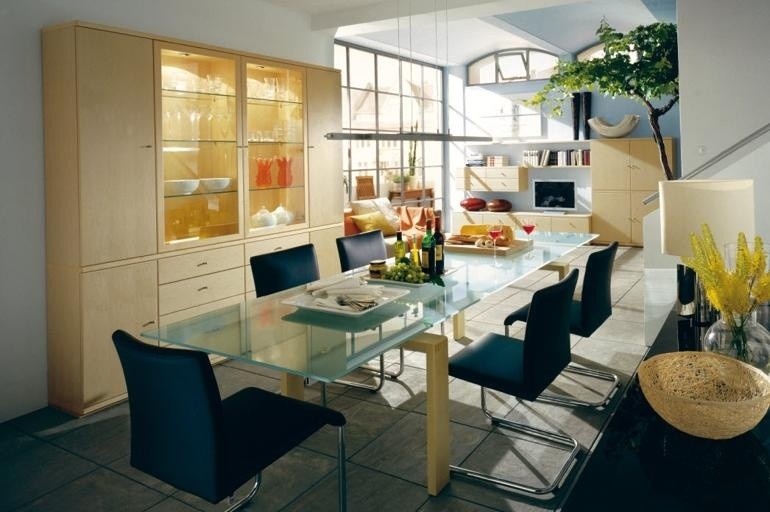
[680,222,770,325]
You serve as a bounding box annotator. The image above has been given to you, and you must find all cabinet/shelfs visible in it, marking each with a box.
[79,260,158,408]
[308,68,344,228]
[591,190,631,246]
[158,243,244,367]
[245,232,309,299]
[464,167,529,193]
[310,228,345,279]
[75,25,158,266]
[630,137,677,190]
[590,139,630,190]
[632,191,659,247]
[153,40,309,254]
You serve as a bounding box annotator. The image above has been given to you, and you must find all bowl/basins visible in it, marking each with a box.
[200,178,232,192]
[638,352,770,439]
[164,179,200,195]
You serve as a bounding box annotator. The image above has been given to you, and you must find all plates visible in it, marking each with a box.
[281,288,410,317]
[361,265,459,288]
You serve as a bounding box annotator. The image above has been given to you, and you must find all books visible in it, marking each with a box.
[523,149,590,166]
[467,153,486,166]
[487,156,510,167]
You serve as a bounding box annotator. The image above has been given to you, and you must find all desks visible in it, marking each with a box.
[555,300,770,511]
[388,187,434,207]
[451,210,591,238]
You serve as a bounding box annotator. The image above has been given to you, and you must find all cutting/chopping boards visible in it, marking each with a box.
[445,238,533,256]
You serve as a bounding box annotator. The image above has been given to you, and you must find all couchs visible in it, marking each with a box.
[345,209,442,250]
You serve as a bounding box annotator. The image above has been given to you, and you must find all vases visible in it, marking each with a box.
[704,318,770,377]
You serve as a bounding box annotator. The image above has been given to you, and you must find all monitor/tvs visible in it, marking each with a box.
[535,182,576,216]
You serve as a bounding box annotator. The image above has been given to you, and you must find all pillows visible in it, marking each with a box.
[350,211,397,237]
[351,197,400,231]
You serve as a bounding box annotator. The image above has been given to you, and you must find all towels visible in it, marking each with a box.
[313,295,353,310]
[337,294,377,311]
[311,276,368,296]
[306,273,345,292]
[326,285,384,297]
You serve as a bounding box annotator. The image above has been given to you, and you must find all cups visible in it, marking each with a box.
[186,102,196,141]
[487,223,502,267]
[204,103,214,140]
[520,217,535,257]
[174,102,183,139]
[166,101,175,139]
[192,102,202,140]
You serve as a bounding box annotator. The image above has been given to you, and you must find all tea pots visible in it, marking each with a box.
[251,206,277,228]
[272,203,289,226]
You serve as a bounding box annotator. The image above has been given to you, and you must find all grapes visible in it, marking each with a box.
[384,262,425,284]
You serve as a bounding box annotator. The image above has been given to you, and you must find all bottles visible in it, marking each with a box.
[410,234,422,267]
[433,218,444,272]
[393,232,405,264]
[421,220,436,276]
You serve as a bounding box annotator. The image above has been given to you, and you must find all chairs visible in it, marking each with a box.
[336,231,389,271]
[447,268,581,494]
[111,329,347,512]
[250,244,404,393]
[504,241,621,408]
